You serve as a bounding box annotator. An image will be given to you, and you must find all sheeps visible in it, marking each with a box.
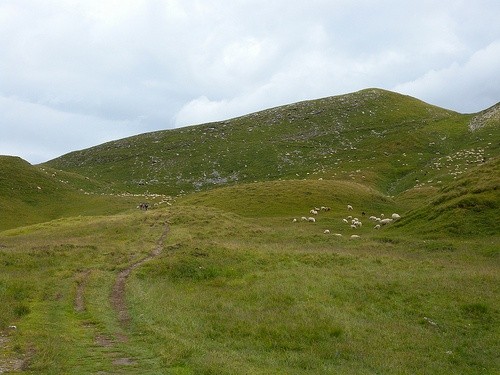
[347,216,352,220]
[36,167,176,209]
[348,205,353,210]
[351,234,361,238]
[323,229,330,234]
[374,224,380,229]
[342,219,348,224]
[425,132,493,186]
[369,215,393,225]
[310,206,330,215]
[392,213,400,219]
[301,217,307,221]
[335,234,342,236]
[308,217,316,223]
[381,214,384,218]
[362,212,366,215]
[350,218,363,229]
[292,219,297,222]
